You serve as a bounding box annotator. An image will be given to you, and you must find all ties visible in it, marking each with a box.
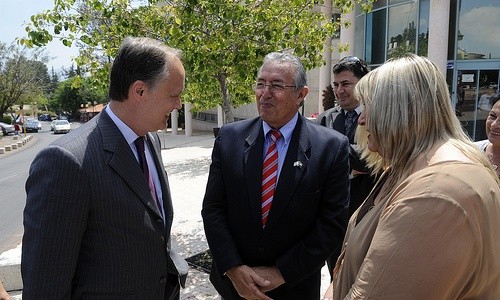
[134,136,157,207]
[345,111,356,136]
[262,130,282,229]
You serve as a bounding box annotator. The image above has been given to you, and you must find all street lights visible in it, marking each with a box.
[81,101,97,117]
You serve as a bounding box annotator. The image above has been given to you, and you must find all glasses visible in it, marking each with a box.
[339,56,366,69]
[252,82,303,92]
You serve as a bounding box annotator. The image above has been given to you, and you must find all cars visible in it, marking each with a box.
[38,114,51,121]
[53,120,72,134]
[50,120,59,131]
[0,122,16,136]
[32,120,41,130]
[22,121,38,133]
[56,116,68,121]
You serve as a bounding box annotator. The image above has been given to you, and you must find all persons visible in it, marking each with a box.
[201,51,351,300]
[478,82,498,110]
[473,96,500,173]
[21,36,186,300]
[318,55,384,282]
[323,51,500,300]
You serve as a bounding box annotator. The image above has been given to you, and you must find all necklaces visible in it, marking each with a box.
[486,146,499,170]
